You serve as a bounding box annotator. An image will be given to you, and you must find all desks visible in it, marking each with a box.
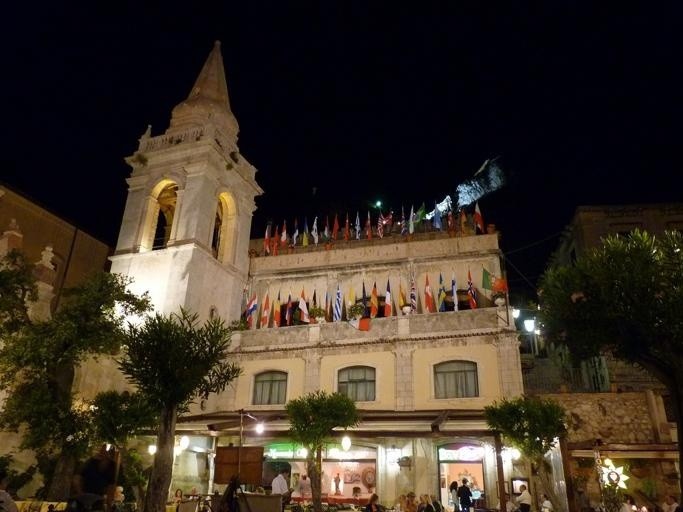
[166,504,178,512]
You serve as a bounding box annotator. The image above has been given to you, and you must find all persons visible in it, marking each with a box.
[71,443,117,512]
[495,485,554,512]
[365,478,472,512]
[664,495,682,512]
[271,462,291,512]
[0,469,18,512]
[174,486,201,512]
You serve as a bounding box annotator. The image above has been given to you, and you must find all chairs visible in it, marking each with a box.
[177,500,367,512]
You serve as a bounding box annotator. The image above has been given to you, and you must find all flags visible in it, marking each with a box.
[263,201,483,256]
[246,266,507,329]
